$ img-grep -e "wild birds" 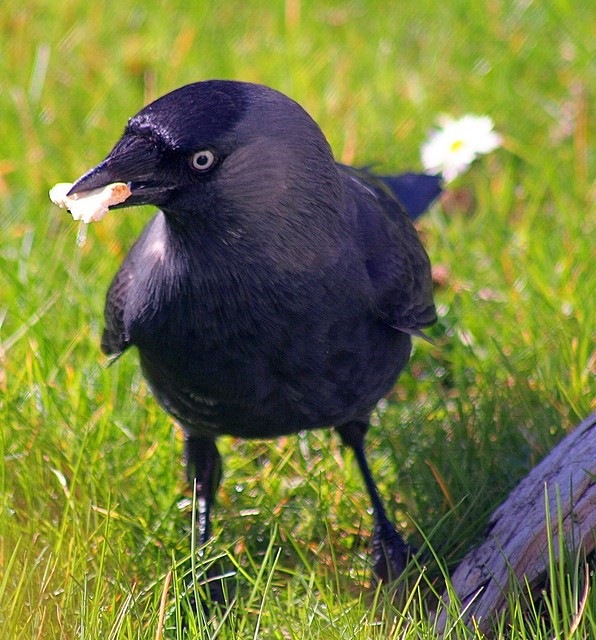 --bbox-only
[66,81,447,640]
